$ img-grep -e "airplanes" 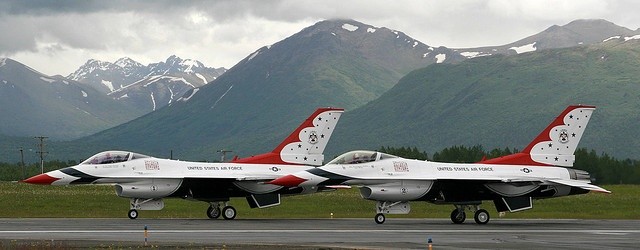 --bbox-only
[22,107,344,220]
[258,106,612,225]
